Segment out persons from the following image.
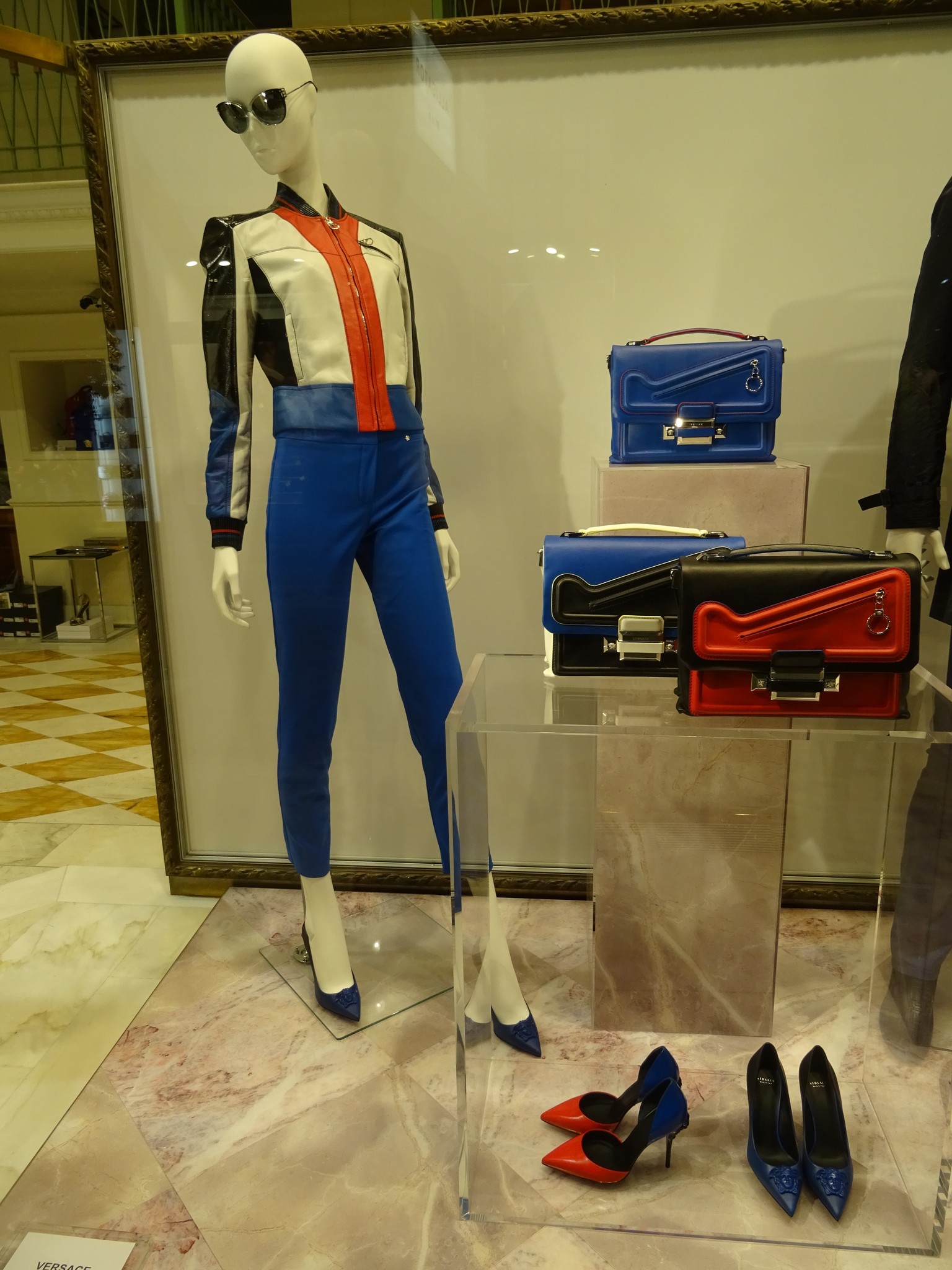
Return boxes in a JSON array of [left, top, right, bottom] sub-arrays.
[[200, 34, 542, 1059], [859, 172, 951, 1053]]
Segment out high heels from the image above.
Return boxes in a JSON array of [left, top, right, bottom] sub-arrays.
[[747, 1043, 799, 1217], [541, 1079, 690, 1186], [799, 1044, 852, 1220], [302, 923, 359, 1022], [541, 1045, 680, 1135], [70, 594, 90, 626], [491, 1001, 542, 1059]]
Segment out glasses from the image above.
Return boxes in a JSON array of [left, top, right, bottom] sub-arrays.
[[218, 81, 318, 134]]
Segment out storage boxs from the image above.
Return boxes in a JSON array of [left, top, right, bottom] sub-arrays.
[[447, 658, 952, 1255], [0, 546, 137, 643]]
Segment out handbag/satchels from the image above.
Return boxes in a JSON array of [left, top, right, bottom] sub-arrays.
[[606, 328, 785, 463], [539, 524, 747, 678], [672, 544, 921, 719]]
